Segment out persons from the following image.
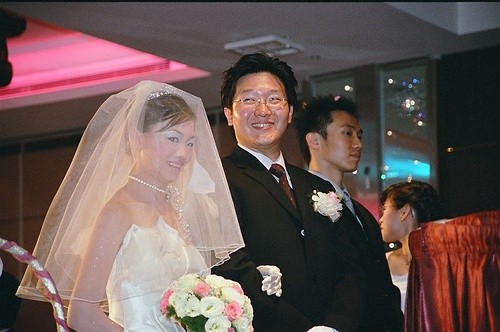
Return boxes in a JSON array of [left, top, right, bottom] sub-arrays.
[[294, 93, 404, 332], [378, 179, 441, 315], [211, 51, 333, 332], [15, 81, 245, 332]]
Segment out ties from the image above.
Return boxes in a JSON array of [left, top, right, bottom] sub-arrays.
[[342, 192, 364, 231], [269, 164, 298, 210]]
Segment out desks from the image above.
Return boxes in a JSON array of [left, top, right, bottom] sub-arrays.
[[405, 210, 500, 332]]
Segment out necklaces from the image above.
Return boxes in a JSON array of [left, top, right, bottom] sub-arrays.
[[127, 176, 167, 193]]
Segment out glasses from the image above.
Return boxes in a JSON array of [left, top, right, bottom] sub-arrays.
[[379, 204, 398, 212], [233, 96, 288, 107]]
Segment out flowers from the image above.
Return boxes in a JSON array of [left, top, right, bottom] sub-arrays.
[[305, 189, 344, 222], [159, 274, 254, 332]]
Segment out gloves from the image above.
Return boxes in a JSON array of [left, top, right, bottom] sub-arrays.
[[256, 265, 283, 298], [307, 325, 338, 332]]
